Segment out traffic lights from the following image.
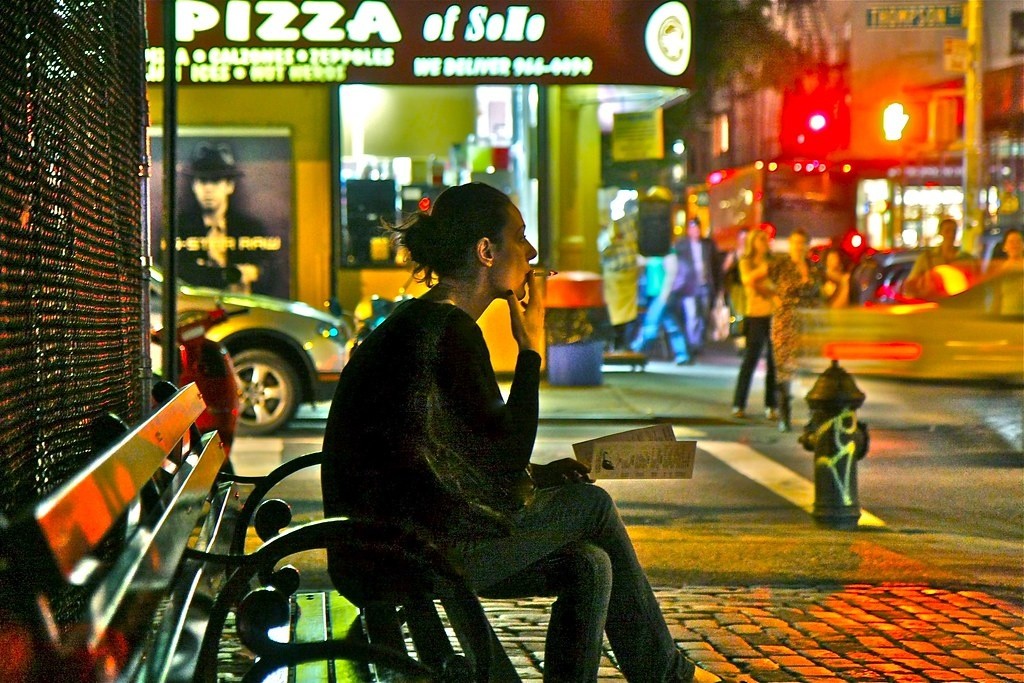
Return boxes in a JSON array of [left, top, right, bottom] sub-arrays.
[[875, 91, 926, 150], [781, 82, 852, 152]]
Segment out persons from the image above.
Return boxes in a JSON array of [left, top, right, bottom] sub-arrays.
[[175, 142, 275, 296], [996, 229, 1024, 316], [321, 183, 723, 683], [748, 229, 845, 431], [731, 228, 777, 419], [823, 249, 860, 309], [601, 198, 748, 365], [902, 219, 965, 296]]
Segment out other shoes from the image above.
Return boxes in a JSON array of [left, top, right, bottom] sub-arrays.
[[765, 409, 778, 420], [733, 407, 744, 417]]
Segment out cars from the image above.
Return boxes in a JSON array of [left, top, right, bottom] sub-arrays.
[[146, 264, 353, 436]]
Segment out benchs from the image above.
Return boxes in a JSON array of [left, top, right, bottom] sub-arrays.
[[12, 382, 493, 683]]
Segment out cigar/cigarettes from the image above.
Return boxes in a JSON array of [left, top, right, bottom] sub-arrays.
[[535, 271, 558, 276]]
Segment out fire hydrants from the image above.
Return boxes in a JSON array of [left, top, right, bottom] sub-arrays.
[[797, 360, 870, 532]]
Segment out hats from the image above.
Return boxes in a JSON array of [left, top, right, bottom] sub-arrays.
[[176, 142, 246, 178]]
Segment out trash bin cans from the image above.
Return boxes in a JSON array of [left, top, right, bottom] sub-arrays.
[[545, 304, 610, 387]]
